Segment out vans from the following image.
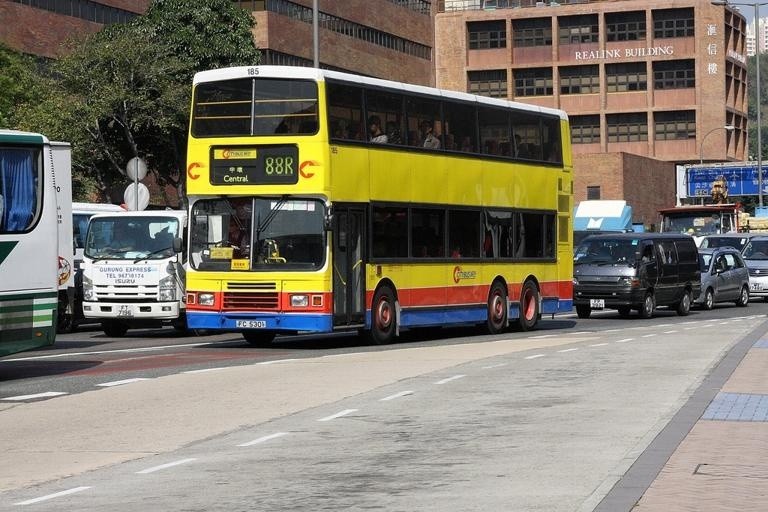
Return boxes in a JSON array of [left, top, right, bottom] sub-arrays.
[[699, 232, 768, 265], [572, 233, 701, 318], [741, 236, 768, 302]]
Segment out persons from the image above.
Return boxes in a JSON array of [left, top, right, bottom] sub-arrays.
[[331, 116, 400, 146], [224, 222, 251, 253], [109, 224, 137, 252], [420, 120, 440, 150]]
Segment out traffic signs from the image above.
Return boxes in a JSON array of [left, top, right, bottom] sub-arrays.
[[742, 167, 768, 195], [688, 168, 741, 197]]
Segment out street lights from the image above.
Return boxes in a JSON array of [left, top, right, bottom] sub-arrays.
[[697, 125, 735, 205], [749, 153, 763, 214], [711, 0, 768, 210]]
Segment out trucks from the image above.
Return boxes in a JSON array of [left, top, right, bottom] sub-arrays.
[[49, 141, 74, 292], [570, 199, 647, 262], [80, 209, 224, 337]]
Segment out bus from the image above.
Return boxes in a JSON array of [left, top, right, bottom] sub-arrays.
[[0, 128, 60, 359], [59, 201, 127, 331], [185, 64, 575, 345]]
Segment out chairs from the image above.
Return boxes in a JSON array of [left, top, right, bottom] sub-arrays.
[[407, 128, 539, 158]]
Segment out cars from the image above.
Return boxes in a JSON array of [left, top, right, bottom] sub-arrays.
[[692, 246, 750, 308]]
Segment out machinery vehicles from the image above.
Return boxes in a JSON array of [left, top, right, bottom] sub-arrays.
[[656, 174, 768, 246]]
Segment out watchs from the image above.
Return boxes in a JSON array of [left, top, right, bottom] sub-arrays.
[[512, 133, 528, 159]]
